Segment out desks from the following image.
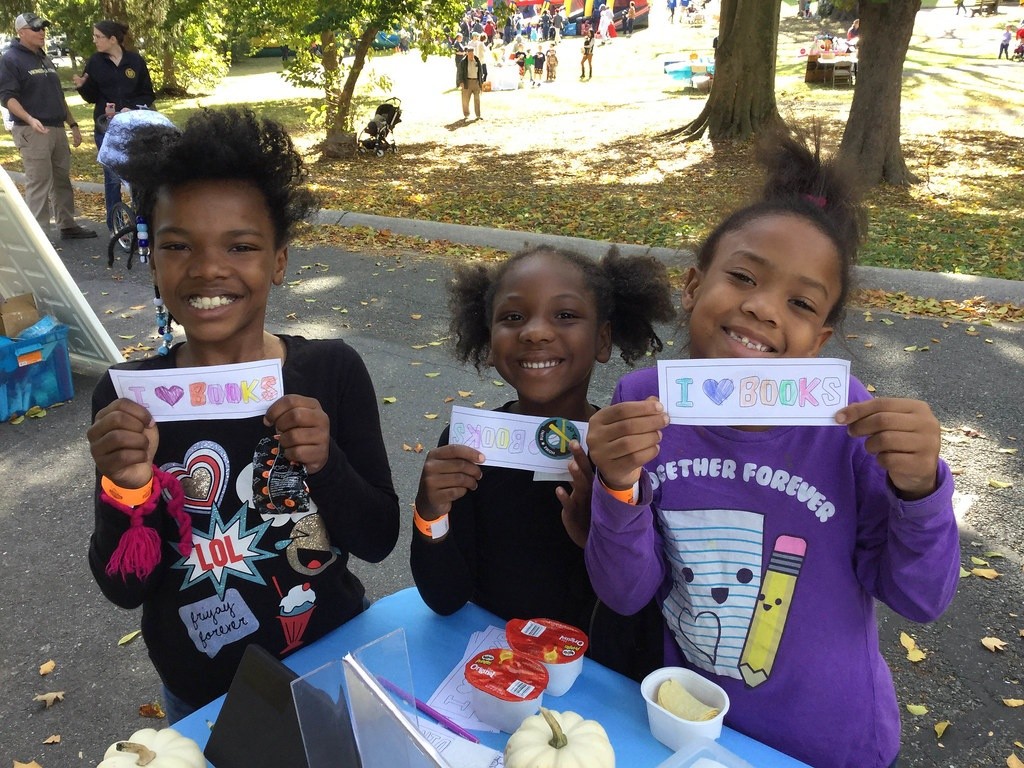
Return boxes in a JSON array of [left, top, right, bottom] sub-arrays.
[[167, 583, 809, 768]]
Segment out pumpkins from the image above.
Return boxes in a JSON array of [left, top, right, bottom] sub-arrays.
[[96, 728, 207, 768], [503, 705, 616, 768]]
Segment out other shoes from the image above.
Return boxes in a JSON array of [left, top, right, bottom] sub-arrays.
[[61, 226, 96, 239]]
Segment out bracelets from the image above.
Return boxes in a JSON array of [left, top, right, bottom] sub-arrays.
[[415, 504, 450, 541], [101, 475, 154, 505], [70, 122, 79, 129], [598, 474, 640, 505]]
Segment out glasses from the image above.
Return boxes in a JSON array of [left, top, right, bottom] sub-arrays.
[[93, 35, 113, 39], [21, 26, 44, 32]]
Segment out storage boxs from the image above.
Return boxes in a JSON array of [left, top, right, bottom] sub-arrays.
[[0, 324, 76, 425], [0, 292, 40, 339]]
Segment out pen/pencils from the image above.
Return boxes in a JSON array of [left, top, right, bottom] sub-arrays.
[[377, 676, 480, 744]]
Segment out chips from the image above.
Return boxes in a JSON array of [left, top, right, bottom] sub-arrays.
[[656, 678, 719, 723]]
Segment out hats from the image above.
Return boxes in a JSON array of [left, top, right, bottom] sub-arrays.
[[15, 13, 50, 32]]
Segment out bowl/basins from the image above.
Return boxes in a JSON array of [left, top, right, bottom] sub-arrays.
[[503, 618, 589, 698], [463, 649, 552, 740], [641, 666, 730, 755]]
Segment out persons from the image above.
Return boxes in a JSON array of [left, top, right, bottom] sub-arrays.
[[805, 1, 811, 17], [584, 122, 962, 768], [73, 21, 157, 239], [847, 19, 859, 79], [410, 245, 678, 682], [88, 108, 402, 725], [454, 2, 636, 120], [0, 13, 98, 241], [667, 0, 700, 24]]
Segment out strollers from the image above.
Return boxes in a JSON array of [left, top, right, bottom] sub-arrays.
[[1011, 39, 1024, 62], [96, 110, 183, 253], [356, 97, 402, 157]]
